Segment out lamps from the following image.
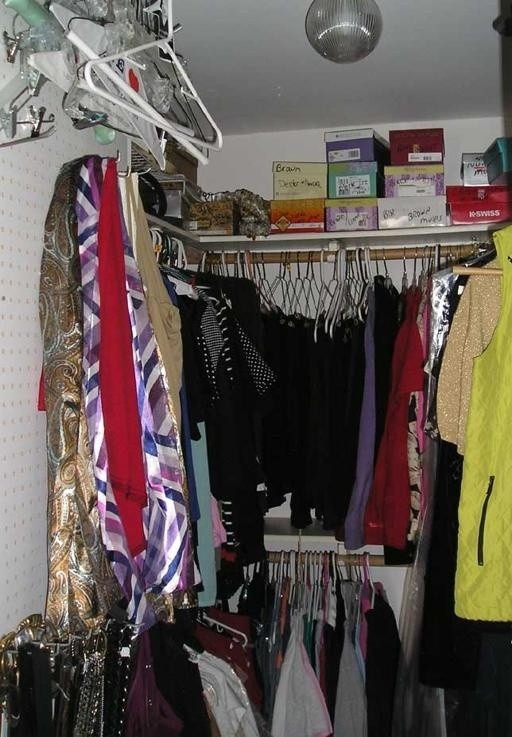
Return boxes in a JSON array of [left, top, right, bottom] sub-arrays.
[[302, 2, 382, 64]]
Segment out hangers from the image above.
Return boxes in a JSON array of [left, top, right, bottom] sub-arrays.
[[260, 541, 373, 665], [1, 591, 249, 675], [17, 0, 222, 164]]
